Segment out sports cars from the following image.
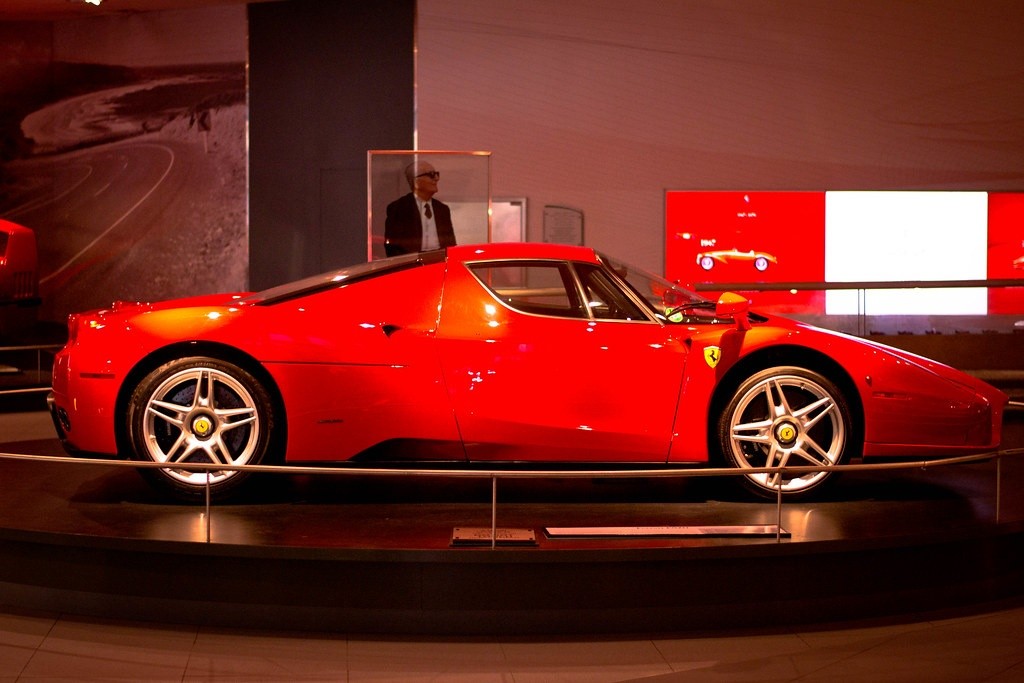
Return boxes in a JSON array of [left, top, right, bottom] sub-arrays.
[[695, 247, 778, 273], [48, 241, 1010, 505]]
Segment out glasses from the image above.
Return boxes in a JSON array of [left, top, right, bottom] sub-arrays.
[[414, 171, 440, 179]]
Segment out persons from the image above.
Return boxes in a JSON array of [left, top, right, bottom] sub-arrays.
[[383, 160, 458, 258]]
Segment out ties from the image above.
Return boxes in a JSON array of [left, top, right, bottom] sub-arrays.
[[423, 203, 432, 219]]
